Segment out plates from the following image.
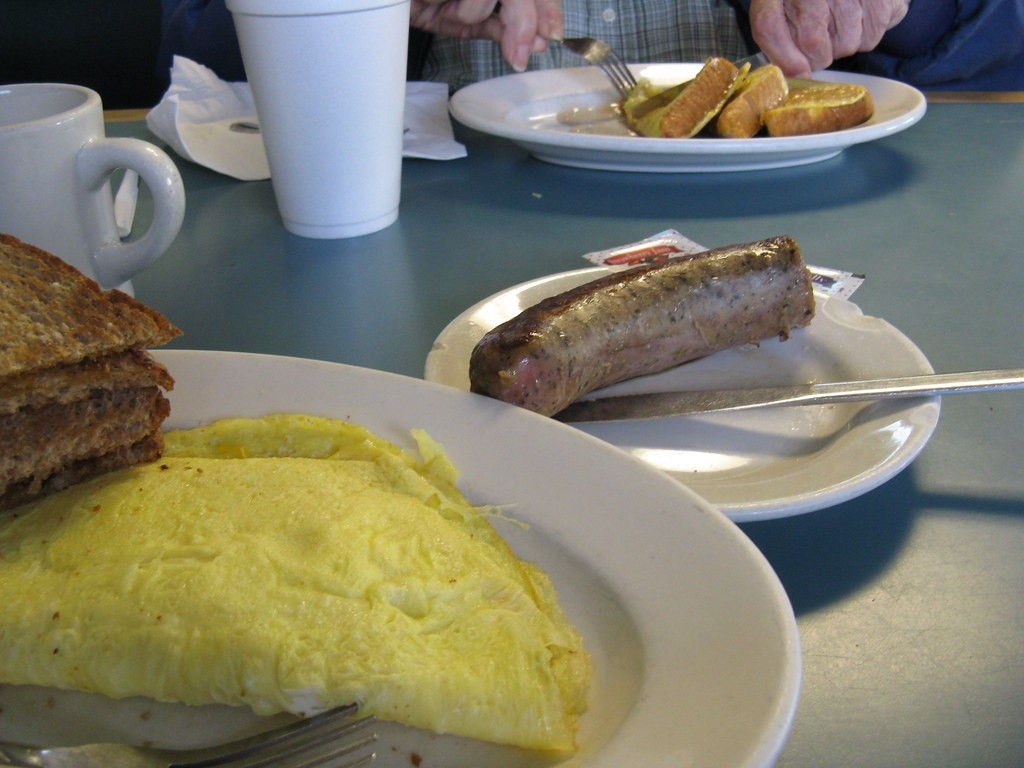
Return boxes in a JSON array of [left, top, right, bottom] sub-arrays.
[[0, 350, 803, 768], [448, 59, 928, 172], [426, 264, 942, 523]]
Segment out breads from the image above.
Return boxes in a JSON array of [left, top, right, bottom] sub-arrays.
[[1, 234, 185, 524]]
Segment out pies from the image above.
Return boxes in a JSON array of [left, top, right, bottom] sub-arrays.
[[1, 413, 593, 753]]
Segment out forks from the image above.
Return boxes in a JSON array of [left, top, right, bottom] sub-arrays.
[[0, 703, 379, 768], [490, 4, 638, 99]]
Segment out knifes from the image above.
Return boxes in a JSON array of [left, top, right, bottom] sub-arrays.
[[551, 365, 1024, 421], [633, 52, 770, 118]]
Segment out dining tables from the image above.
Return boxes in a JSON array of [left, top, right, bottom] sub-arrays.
[[0, 90, 1022, 768]]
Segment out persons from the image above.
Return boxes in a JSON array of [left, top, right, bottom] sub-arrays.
[[139, 0, 1024, 106]]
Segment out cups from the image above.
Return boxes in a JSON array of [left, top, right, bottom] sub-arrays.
[[1, 83, 182, 327], [226, 0, 415, 237]]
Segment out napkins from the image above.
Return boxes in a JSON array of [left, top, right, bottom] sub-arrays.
[[145, 51, 467, 180]]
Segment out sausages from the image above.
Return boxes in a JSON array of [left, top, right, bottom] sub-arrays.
[[467, 233, 816, 418]]
